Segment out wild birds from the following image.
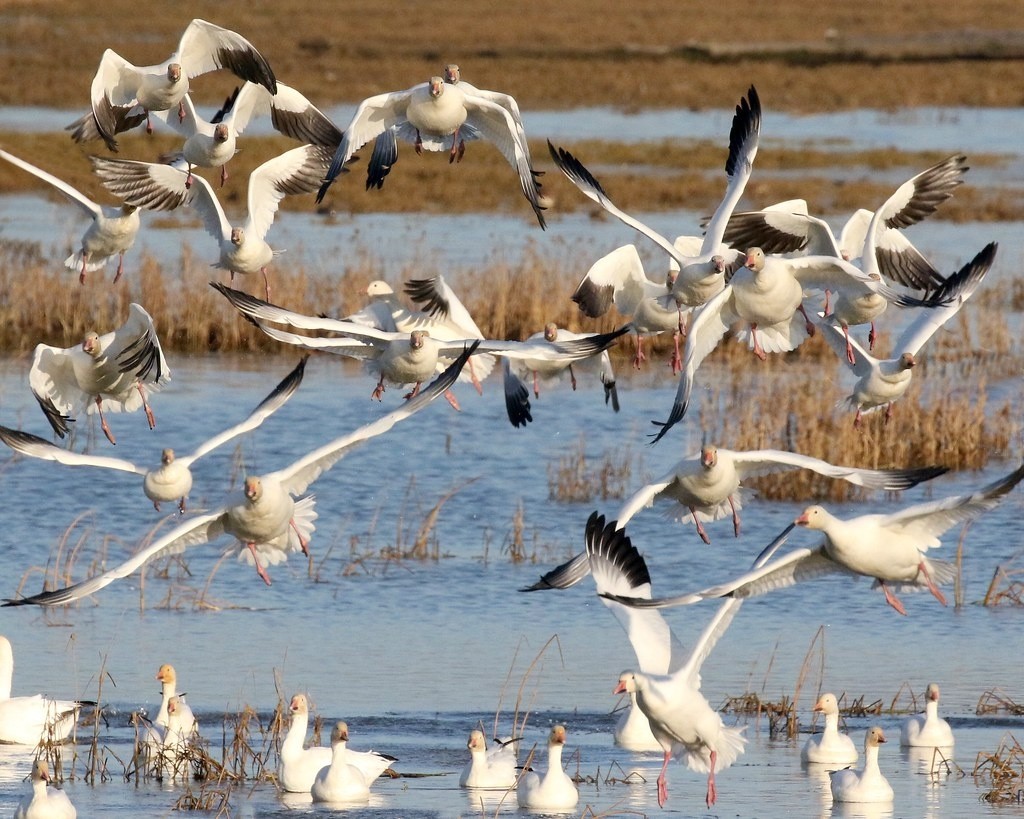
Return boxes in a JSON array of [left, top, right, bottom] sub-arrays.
[[2, 19, 1023, 615], [279, 693, 401, 804], [827, 726, 894, 804], [141, 663, 204, 779], [899, 682, 955, 747], [584, 511, 805, 810], [513, 723, 578, 810], [801, 691, 859, 766], [458, 730, 525, 791], [13, 759, 76, 819], [0, 636, 98, 748]]
